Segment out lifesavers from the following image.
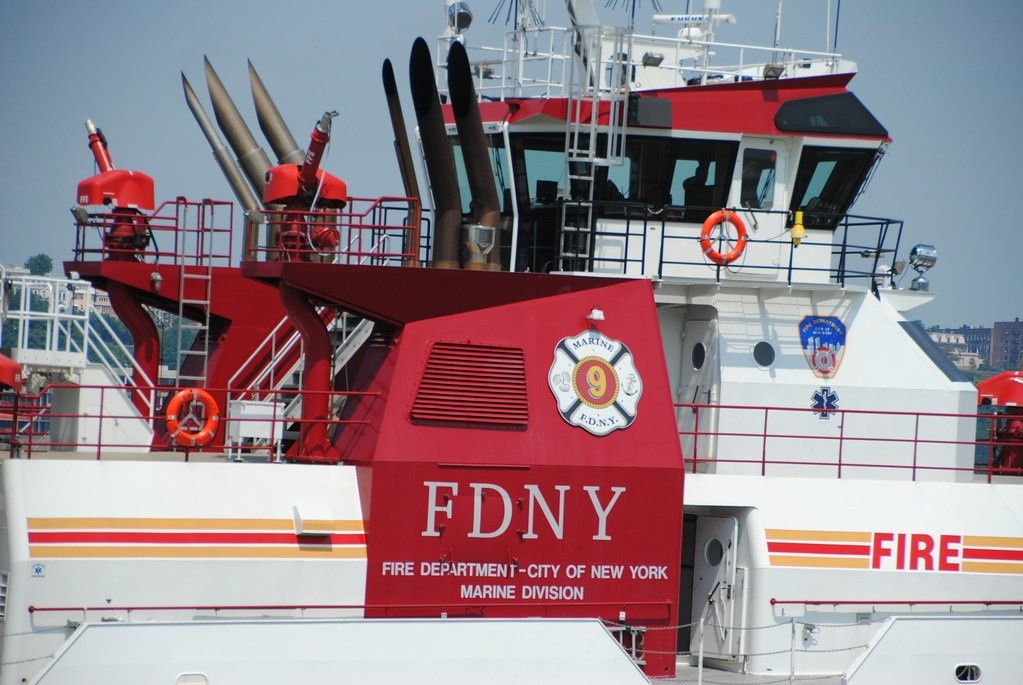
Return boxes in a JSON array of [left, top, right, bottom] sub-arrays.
[[167, 389, 220, 446], [701, 208, 747, 265]]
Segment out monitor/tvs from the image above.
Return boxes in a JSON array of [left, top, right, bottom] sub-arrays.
[[537, 180, 558, 204]]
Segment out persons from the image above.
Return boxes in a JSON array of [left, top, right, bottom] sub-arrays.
[[1000, 407, 1023, 468]]
